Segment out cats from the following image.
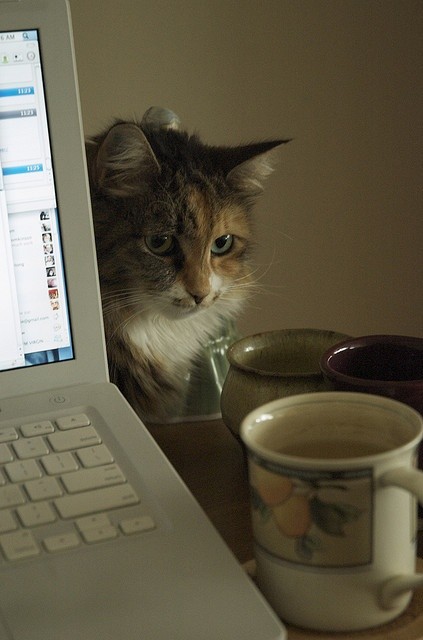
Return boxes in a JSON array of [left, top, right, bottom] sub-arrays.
[[84, 116, 291, 433]]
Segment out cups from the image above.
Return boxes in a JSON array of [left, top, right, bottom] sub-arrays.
[[318, 333, 422, 421], [239, 392, 422, 634], [218, 327, 357, 454]]
